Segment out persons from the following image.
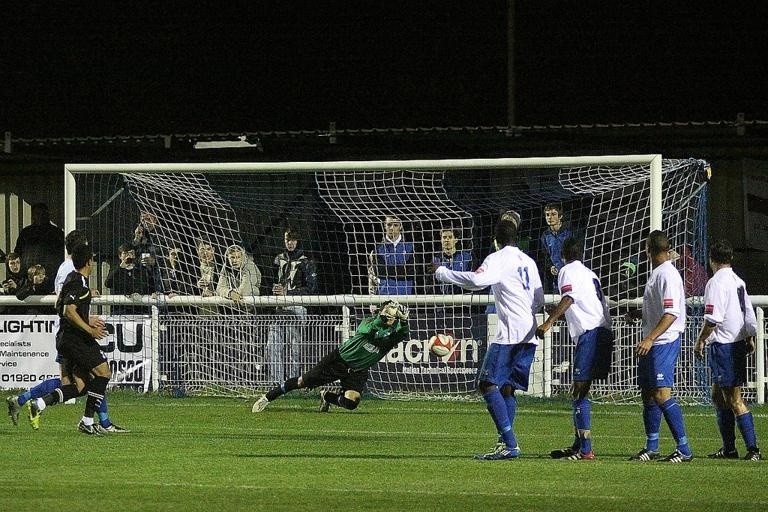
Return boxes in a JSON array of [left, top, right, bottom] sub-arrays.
[[535, 236, 612, 460], [542, 202, 583, 392], [369, 215, 419, 391], [470, 210, 530, 397], [7, 231, 131, 434], [1, 204, 262, 385], [694, 240, 761, 462], [630, 230, 693, 463], [433, 220, 544, 459], [674, 236, 710, 401], [431, 228, 473, 389], [28, 247, 110, 436], [268, 231, 317, 387], [253, 301, 408, 413]]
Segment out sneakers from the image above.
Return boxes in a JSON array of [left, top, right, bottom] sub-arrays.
[[563, 451, 596, 461], [657, 451, 694, 463], [77, 419, 107, 435], [27, 397, 42, 430], [319, 390, 330, 413], [551, 446, 578, 457], [104, 424, 130, 433], [627, 448, 660, 461], [474, 442, 522, 461], [7, 394, 21, 426], [706, 448, 739, 459], [252, 394, 270, 413], [745, 449, 761, 461]]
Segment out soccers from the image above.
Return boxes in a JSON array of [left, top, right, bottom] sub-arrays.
[[428, 334, 451, 357]]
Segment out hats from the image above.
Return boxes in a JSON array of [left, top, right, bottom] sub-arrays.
[[284, 231, 300, 240], [501, 210, 520, 231]]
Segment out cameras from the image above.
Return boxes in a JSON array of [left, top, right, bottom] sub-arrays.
[[3, 280, 10, 287]]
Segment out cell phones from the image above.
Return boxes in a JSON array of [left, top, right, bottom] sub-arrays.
[[125, 258, 138, 264]]
